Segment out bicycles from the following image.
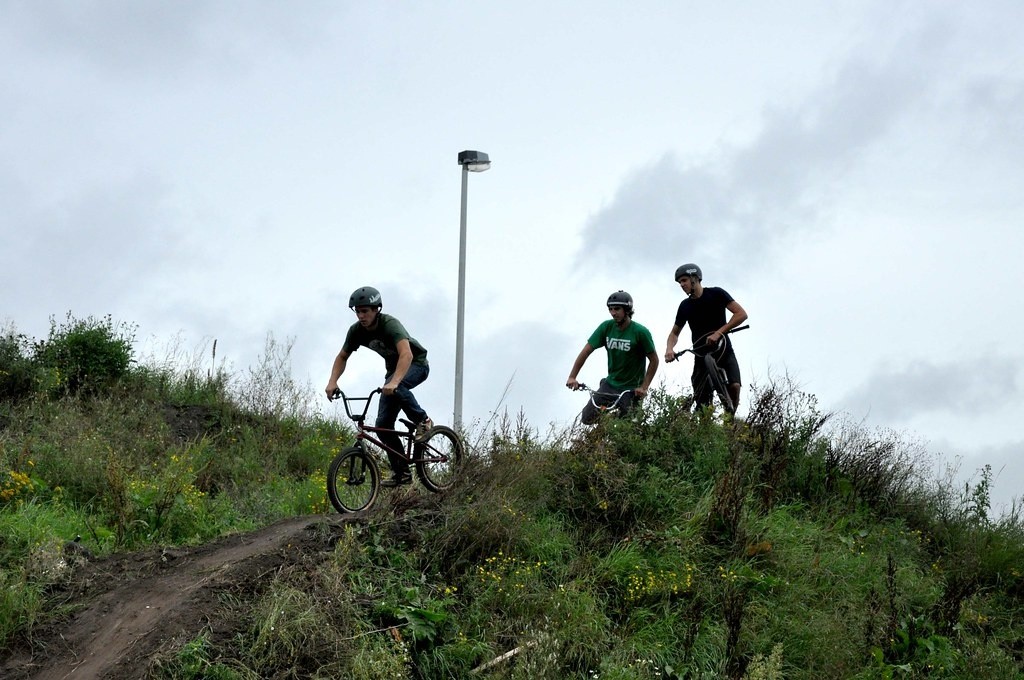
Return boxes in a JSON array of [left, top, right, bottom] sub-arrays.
[[567, 382, 641, 433], [673, 322, 751, 415], [325, 387, 466, 513]]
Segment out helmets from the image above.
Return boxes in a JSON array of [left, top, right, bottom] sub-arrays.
[[607, 291, 634, 308], [674, 264, 702, 282], [349, 286, 382, 312]]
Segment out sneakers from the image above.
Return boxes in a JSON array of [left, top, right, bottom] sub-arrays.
[[413, 417, 434, 444], [380, 469, 414, 488]]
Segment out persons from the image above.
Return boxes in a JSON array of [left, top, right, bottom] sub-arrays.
[[664, 264, 748, 426], [566, 290, 659, 425], [324, 286, 434, 487]]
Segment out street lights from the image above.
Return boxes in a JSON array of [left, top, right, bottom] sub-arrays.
[[450, 150, 493, 444]]
